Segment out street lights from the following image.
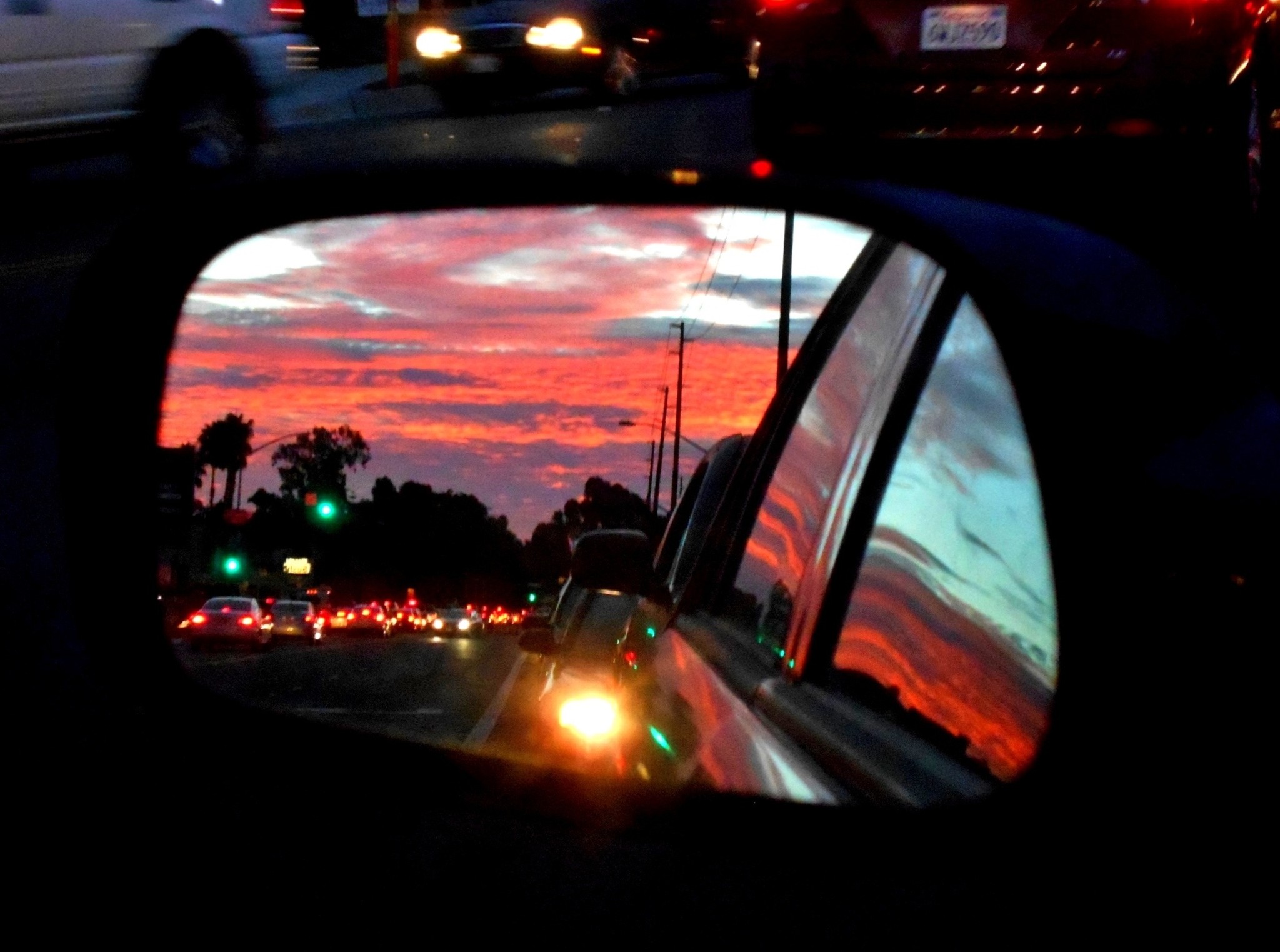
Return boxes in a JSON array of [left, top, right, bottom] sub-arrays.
[[236, 431, 309, 519]]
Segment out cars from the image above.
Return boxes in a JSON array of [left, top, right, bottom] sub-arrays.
[[319, 603, 527, 640], [531, 576, 633, 779], [190, 597, 264, 646], [403, 1, 764, 119], [1, 0, 321, 145], [268, 600, 319, 643]]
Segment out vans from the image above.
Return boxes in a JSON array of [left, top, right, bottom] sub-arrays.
[[568, 526, 653, 595]]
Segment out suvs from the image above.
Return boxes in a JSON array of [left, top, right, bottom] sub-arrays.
[[899, 3, 1278, 203]]
